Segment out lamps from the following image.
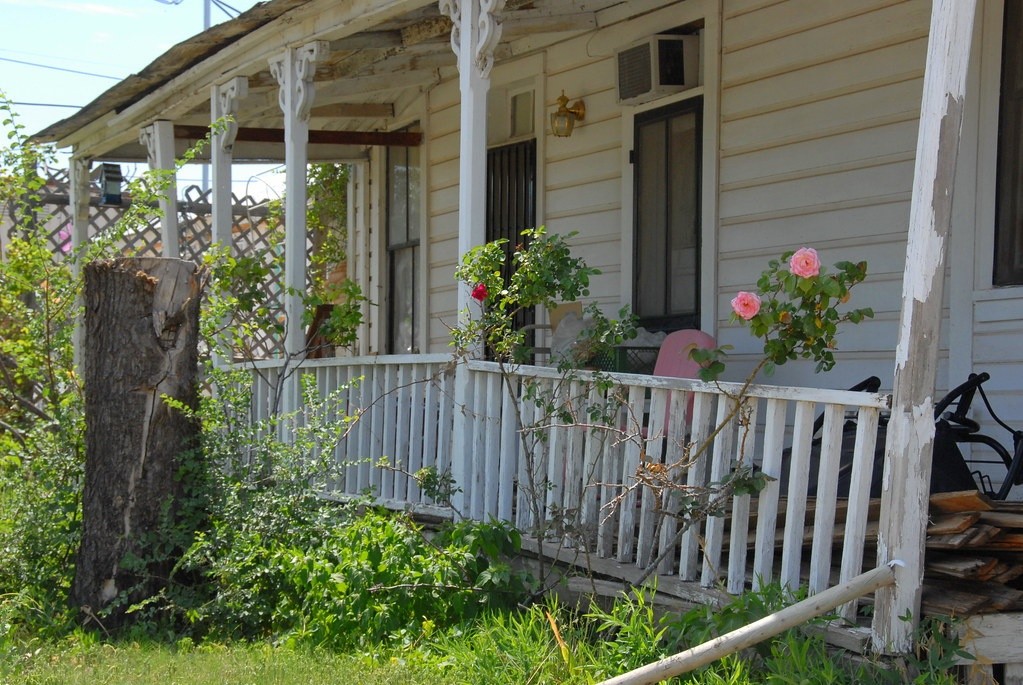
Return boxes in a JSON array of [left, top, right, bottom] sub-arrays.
[[549, 91, 586, 137]]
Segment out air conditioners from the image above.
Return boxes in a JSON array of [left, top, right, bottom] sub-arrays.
[[614, 34, 700, 106]]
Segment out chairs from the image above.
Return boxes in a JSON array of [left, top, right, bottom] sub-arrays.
[[582, 327, 717, 449]]
